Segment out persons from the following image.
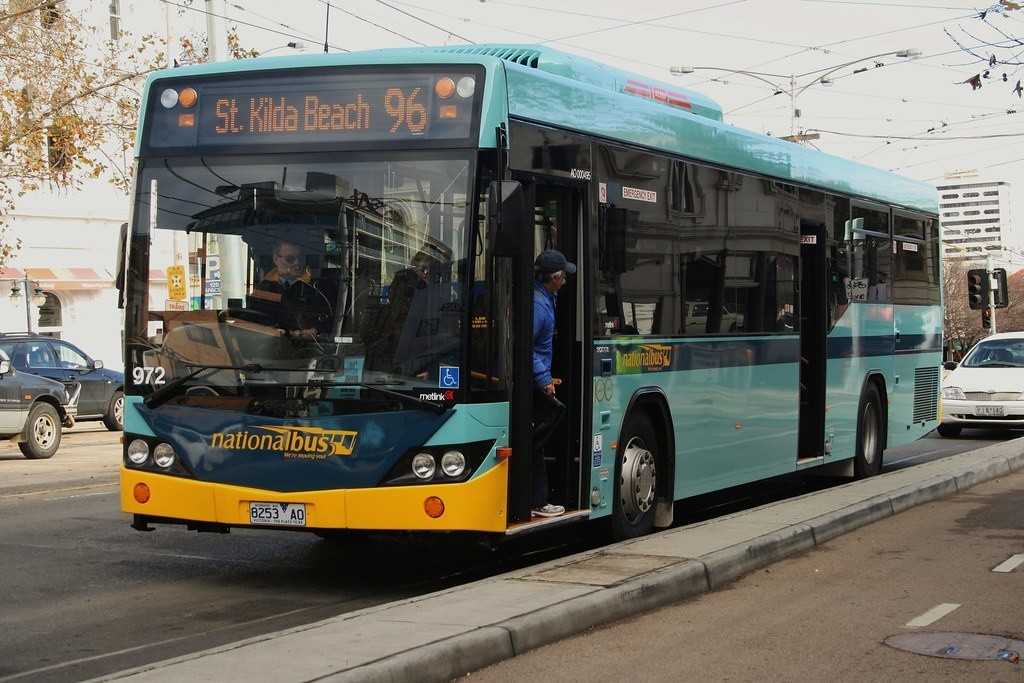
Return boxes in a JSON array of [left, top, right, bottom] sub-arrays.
[[242, 237, 339, 341], [985, 348, 1008, 363], [529, 248, 577, 518]]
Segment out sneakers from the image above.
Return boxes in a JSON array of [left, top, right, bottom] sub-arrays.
[[530, 503, 565, 517]]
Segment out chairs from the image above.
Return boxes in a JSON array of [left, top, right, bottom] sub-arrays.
[[29, 351, 50, 367], [303, 267, 341, 333]]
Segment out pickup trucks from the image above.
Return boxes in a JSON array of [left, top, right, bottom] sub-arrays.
[[0, 347, 76, 460]]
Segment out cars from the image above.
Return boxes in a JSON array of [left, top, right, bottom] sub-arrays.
[[0, 330, 124, 430], [935, 330, 1024, 436]]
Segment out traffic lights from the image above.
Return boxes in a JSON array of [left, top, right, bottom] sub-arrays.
[[982, 308, 990, 327], [966, 269, 990, 310]]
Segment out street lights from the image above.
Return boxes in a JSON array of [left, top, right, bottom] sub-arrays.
[[669, 44, 924, 144], [8, 269, 48, 334]]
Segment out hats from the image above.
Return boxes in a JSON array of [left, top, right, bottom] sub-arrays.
[[534, 250, 576, 275]]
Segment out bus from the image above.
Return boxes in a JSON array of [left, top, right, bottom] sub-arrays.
[[115, 45, 945, 569]]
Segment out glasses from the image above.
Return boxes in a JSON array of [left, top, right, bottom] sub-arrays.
[[277, 255, 307, 264]]
